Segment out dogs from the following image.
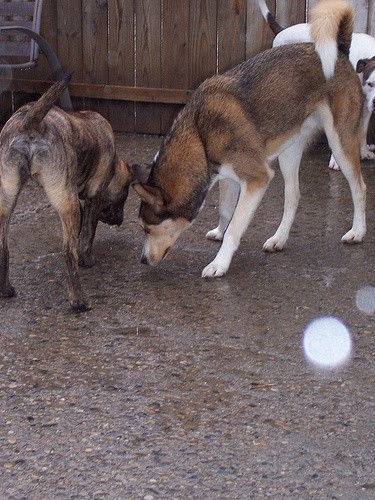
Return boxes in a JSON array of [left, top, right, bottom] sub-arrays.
[[259, 0, 375, 171], [0, 73, 136, 314], [129, 0, 368, 278]]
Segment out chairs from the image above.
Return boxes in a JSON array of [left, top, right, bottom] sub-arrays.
[[0, 0, 74, 113]]
